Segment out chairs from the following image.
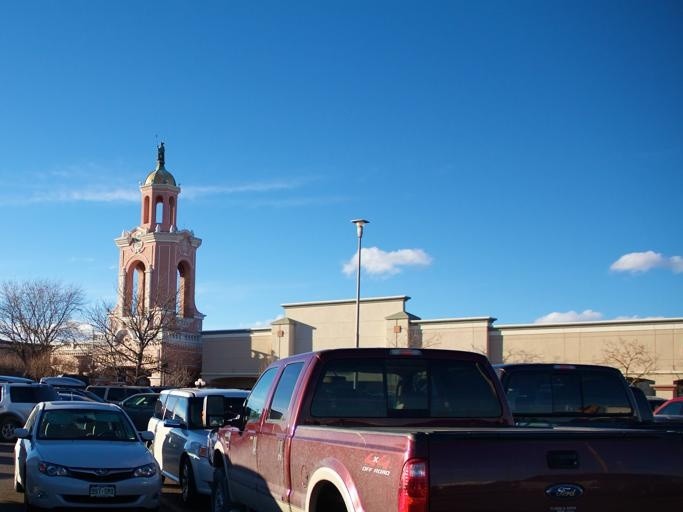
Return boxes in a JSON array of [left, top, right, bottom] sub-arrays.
[[43, 414, 80, 438]]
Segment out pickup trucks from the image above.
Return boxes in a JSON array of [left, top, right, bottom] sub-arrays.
[[208, 346, 681, 512]]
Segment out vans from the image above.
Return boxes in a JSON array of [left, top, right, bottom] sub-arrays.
[[146, 379, 253, 508]]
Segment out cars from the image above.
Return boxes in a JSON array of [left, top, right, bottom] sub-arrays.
[[490, 362, 683, 431], [13, 400, 164, 512], [1, 373, 161, 441]]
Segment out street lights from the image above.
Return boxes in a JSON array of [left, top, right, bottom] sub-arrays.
[[350, 217, 371, 349]]
